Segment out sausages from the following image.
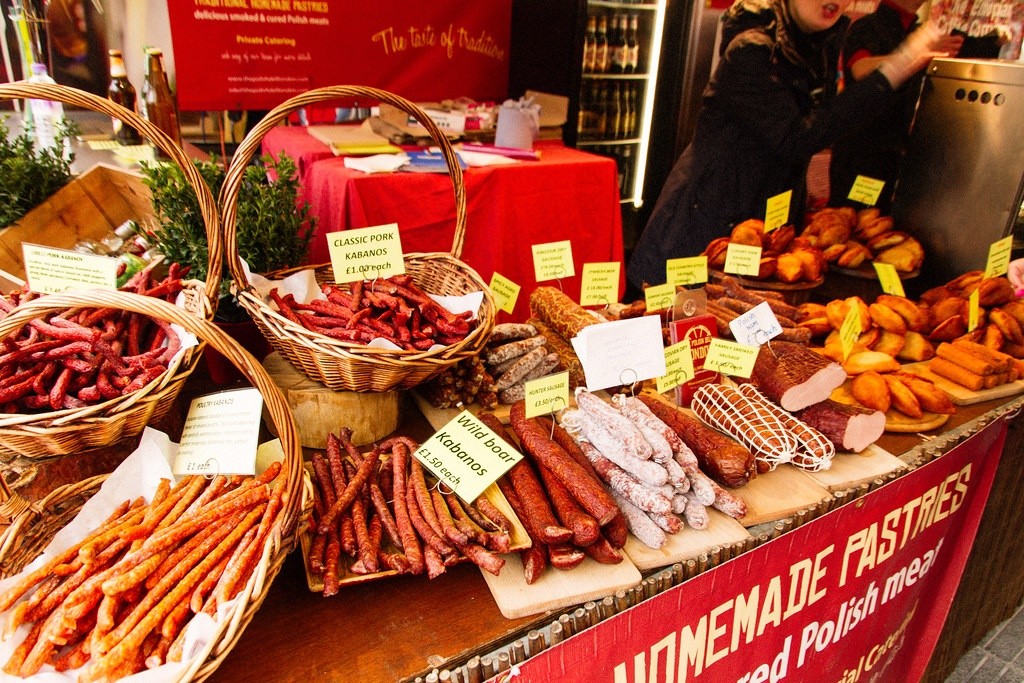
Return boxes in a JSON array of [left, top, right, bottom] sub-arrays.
[[2, 252, 886, 681]]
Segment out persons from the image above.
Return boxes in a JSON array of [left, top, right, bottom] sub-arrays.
[[644, 1, 948, 297]]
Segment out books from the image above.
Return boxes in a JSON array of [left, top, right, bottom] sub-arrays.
[[398, 152, 469, 173], [305, 124, 403, 156]]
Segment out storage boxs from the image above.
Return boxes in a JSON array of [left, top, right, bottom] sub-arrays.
[[0, 162, 196, 298]]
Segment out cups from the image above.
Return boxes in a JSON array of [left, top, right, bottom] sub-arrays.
[[496, 105, 534, 152]]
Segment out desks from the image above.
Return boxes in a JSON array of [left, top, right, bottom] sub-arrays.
[[0, 307, 1023, 683], [261, 124, 469, 218], [290, 142, 626, 323]]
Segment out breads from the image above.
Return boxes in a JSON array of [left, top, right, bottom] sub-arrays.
[[697, 206, 1023, 418]]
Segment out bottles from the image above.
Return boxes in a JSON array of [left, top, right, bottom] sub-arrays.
[[72, 216, 160, 285], [139, 43, 185, 162], [26, 62, 74, 155], [577, 78, 640, 140], [107, 49, 141, 146], [577, 5, 641, 73], [591, 149, 632, 200]]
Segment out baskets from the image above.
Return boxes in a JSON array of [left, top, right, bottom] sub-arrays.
[[218, 83, 499, 395], [0, 81, 224, 462], [0, 289, 315, 683]]
